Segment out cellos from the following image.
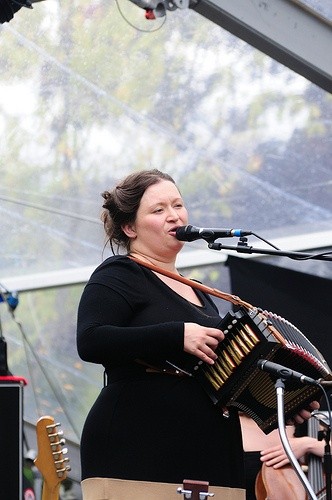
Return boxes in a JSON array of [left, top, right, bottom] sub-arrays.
[[253, 406, 332, 500]]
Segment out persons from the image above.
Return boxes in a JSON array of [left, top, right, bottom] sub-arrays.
[[77, 168, 320, 500], [258, 434, 332, 470]]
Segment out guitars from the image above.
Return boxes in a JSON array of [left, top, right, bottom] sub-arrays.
[[32, 416, 71, 499]]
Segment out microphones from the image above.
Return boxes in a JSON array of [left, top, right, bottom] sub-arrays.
[[175, 225, 253, 241], [256, 358, 316, 385]]
